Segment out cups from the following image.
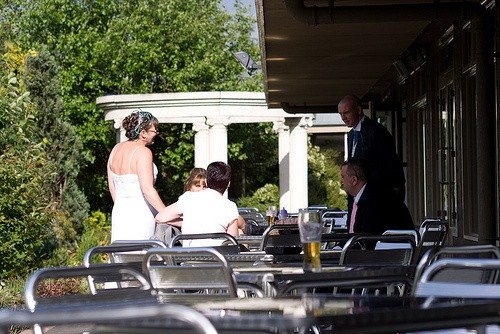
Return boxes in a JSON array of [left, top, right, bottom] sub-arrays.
[[266, 210, 276, 226], [298, 208, 323, 273]]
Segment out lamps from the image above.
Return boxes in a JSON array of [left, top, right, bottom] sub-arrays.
[[232, 52, 263, 75], [393, 60, 416, 83]]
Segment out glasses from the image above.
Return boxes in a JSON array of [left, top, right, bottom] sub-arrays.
[[146, 129, 159, 135]]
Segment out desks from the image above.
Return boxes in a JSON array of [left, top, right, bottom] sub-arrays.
[[165, 294, 414, 316], [235, 232, 363, 250], [237, 267, 410, 286]]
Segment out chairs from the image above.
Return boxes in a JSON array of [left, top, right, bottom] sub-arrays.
[[0, 205, 500, 334]]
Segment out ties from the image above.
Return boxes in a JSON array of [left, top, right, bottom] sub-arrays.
[[348, 201, 359, 233], [352, 130, 359, 158]]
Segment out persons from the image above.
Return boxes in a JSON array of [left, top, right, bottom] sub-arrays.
[[339, 95, 395, 195], [327, 159, 416, 248], [107, 111, 166, 254], [154, 162, 246, 248]]
